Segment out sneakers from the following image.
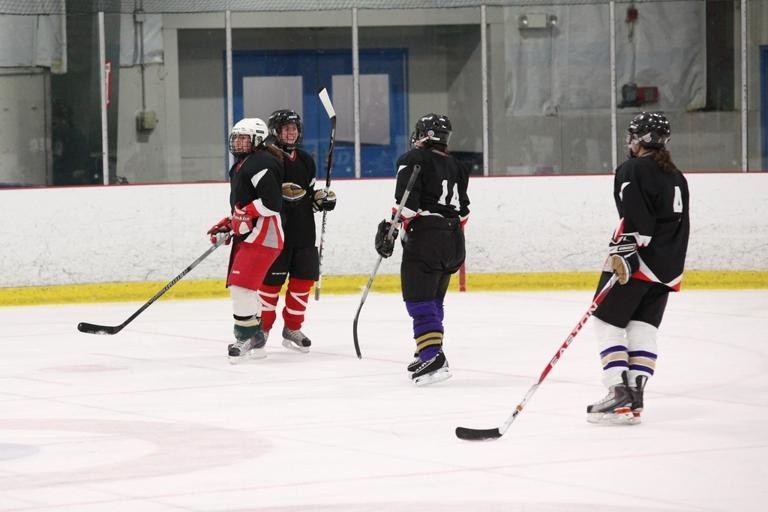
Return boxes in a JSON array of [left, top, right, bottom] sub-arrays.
[[630, 387, 644, 413], [252, 330, 269, 347], [407, 354, 424, 372], [588, 384, 631, 412], [412, 349, 449, 379], [282, 326, 311, 347], [228, 337, 255, 356]]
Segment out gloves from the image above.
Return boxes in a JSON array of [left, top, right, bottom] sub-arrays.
[[207, 217, 233, 246], [610, 234, 640, 273], [282, 183, 307, 202], [231, 202, 253, 236], [311, 189, 336, 213], [375, 219, 398, 258]]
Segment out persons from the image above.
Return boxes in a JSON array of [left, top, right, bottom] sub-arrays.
[[206, 117, 286, 364], [586, 112, 690, 425], [375, 111, 471, 385], [254, 109, 336, 352]]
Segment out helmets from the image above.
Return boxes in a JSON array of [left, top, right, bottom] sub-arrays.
[[624, 112, 671, 158], [409, 114, 453, 149], [228, 117, 269, 158], [267, 110, 303, 151]]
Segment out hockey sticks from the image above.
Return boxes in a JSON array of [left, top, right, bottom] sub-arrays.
[[354, 165, 420, 358], [314, 88, 336, 300], [455, 273, 619, 441], [77, 232, 230, 334]]
